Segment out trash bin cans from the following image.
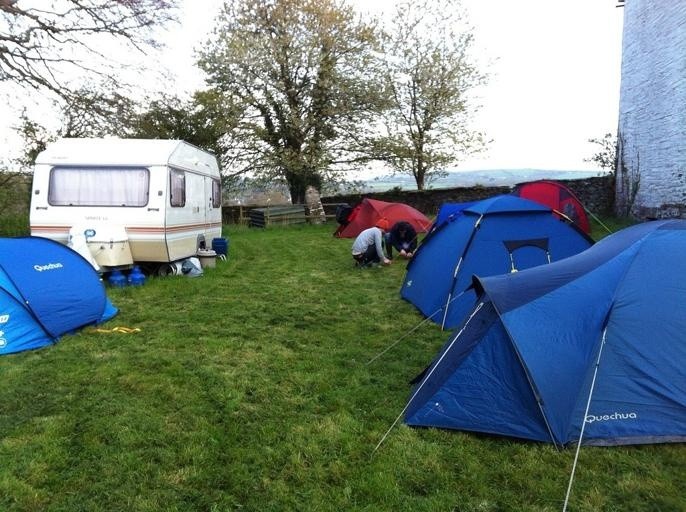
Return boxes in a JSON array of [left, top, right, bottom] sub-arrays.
[[196, 237, 228, 269]]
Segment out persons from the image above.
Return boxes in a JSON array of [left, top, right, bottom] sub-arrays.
[[384, 221, 418, 258], [352, 225, 391, 267]]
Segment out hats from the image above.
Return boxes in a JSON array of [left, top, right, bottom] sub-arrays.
[[376, 218, 389, 230]]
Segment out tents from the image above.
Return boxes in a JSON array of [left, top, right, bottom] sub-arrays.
[[332, 198, 433, 238], [398, 194, 594, 331], [436, 199, 479, 227], [405, 216, 686, 452], [519, 179, 591, 234], [1, 235, 120, 357]]
